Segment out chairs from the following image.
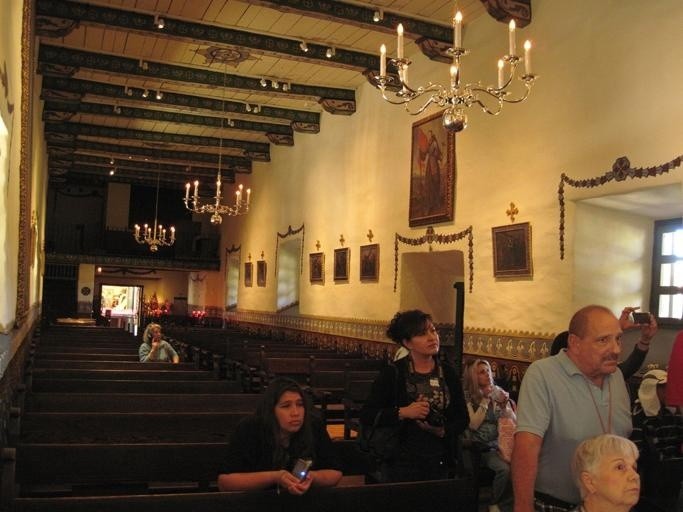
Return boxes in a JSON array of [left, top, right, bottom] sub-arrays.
[[627, 412, 683, 512]]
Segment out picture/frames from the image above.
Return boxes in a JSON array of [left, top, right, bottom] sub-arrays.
[[408, 106, 456, 226], [492, 222, 533, 278], [309, 252, 325, 281], [245, 261, 254, 287], [256, 260, 267, 287], [334, 247, 350, 280], [360, 244, 380, 280]]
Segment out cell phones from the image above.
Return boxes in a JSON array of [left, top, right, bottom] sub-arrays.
[[632, 309, 652, 328]]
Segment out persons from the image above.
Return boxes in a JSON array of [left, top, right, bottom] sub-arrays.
[[457, 359, 517, 512], [572, 434, 642, 512], [216, 377, 342, 498], [665, 330, 682, 417], [418, 129, 443, 216], [510, 303, 634, 511], [139, 322, 180, 363], [356, 309, 470, 485], [549, 305, 659, 382]]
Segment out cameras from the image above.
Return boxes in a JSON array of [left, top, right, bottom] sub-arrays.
[[288, 458, 314, 478]]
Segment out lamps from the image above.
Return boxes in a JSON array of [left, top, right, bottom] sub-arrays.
[[109, 155, 118, 177], [325, 46, 335, 58], [374, 1, 541, 134], [300, 41, 308, 53], [132, 158, 177, 253], [182, 54, 252, 225], [373, 10, 382, 22], [228, 78, 290, 127], [112, 15, 168, 115]]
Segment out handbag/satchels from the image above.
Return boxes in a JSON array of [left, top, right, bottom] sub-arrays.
[[496, 419, 517, 462]]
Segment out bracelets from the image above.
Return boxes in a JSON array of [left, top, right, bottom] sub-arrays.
[[479, 403, 488, 411], [639, 339, 650, 346]]
